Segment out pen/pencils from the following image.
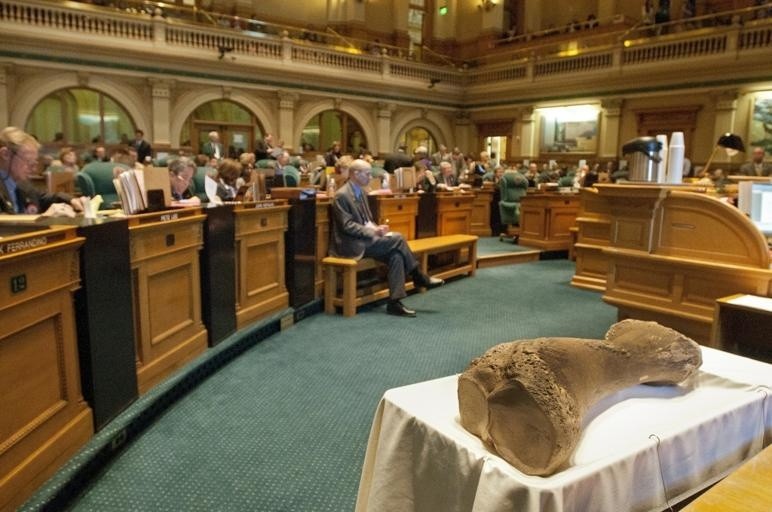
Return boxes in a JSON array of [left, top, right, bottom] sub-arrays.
[[384, 219, 390, 225]]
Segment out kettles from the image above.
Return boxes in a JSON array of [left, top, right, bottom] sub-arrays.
[[620, 136, 663, 184]]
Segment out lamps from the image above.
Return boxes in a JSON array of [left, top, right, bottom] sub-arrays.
[[697, 133, 745, 177]]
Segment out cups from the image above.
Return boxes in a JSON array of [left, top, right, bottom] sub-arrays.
[[82, 200, 100, 217], [654, 130, 685, 184]]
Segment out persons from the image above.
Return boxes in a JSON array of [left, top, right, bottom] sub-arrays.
[[355, 144, 601, 188], [331, 159, 446, 318], [640, 0, 772, 43], [500, 14, 597, 44], [247, 14, 263, 52], [0, 126, 355, 219], [301, 23, 319, 43], [741, 146, 772, 176], [366, 38, 382, 54]]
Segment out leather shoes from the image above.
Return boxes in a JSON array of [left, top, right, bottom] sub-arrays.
[[414, 271, 445, 288], [386, 300, 417, 316]]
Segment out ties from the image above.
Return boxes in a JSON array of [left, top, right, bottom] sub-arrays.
[[359, 194, 370, 222]]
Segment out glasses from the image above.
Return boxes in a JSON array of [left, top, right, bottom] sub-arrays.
[[13, 151, 39, 166]]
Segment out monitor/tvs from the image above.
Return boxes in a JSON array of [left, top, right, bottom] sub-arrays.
[[752, 184, 772, 249]]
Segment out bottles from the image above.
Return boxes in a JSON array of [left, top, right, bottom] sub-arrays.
[[328, 178, 336, 194]]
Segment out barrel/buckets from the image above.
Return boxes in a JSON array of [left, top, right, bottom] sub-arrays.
[[627, 138, 663, 181]]
